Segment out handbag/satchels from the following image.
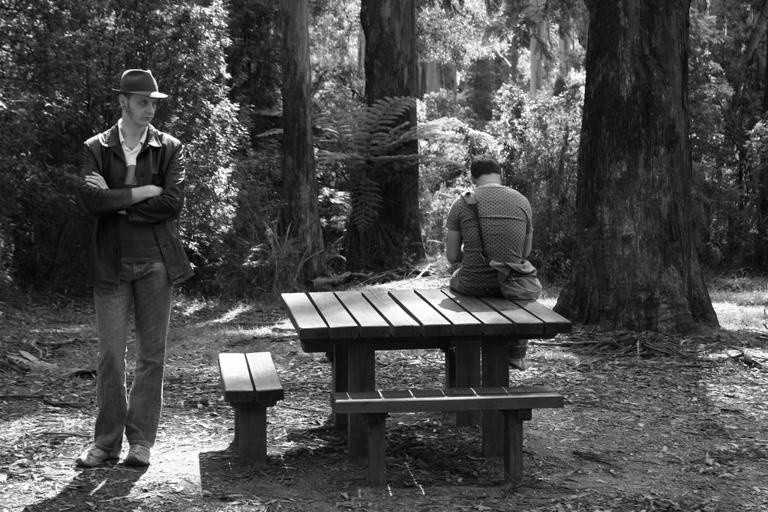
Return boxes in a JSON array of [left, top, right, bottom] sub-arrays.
[[489, 259, 542, 300]]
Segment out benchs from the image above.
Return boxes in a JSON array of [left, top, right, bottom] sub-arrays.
[[218, 351, 284, 467], [331, 386, 564, 487]]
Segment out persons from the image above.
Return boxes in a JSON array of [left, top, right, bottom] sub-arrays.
[[445, 148, 542, 369], [69, 69, 191, 471]]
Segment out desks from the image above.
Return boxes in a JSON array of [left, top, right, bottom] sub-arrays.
[[281, 288, 571, 466]]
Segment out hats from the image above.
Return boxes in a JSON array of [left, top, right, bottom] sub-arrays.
[[112, 69, 169, 99]]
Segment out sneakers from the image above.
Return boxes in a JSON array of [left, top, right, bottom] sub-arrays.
[[76, 446, 119, 466], [509, 357, 527, 371], [127, 445, 151, 465]]
